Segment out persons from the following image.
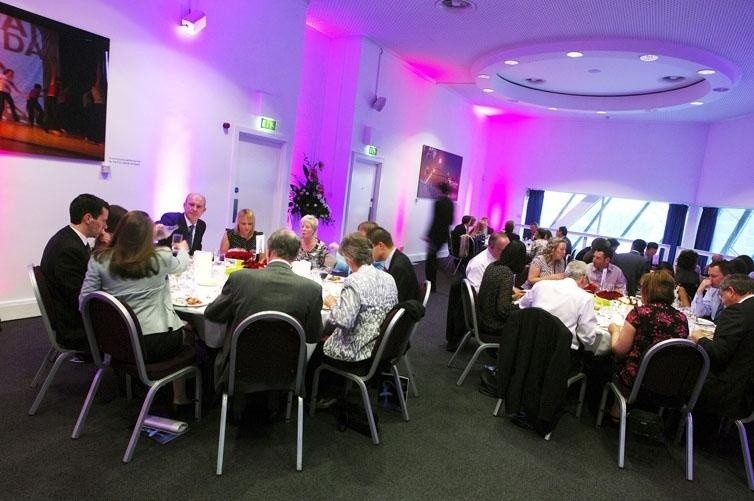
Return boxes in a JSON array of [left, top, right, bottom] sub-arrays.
[[202, 229, 325, 427], [306, 230, 401, 409], [154, 191, 208, 258], [218, 207, 264, 259], [419, 179, 458, 297], [447, 214, 754, 431], [368, 226, 421, 307], [1, 59, 105, 141], [291, 213, 328, 269], [76, 210, 198, 410], [36, 191, 112, 354], [102, 203, 168, 239], [324, 221, 387, 276]]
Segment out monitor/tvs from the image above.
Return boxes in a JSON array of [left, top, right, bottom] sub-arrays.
[[0, 2, 110, 161]]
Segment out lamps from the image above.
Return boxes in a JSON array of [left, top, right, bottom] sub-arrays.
[[178, 0, 206, 36]]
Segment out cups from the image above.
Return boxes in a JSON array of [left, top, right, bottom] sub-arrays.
[[214, 254, 225, 268], [171, 234, 181, 253], [331, 264, 342, 282]]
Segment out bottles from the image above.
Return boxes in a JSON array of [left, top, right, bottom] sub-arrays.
[[154, 225, 177, 241]]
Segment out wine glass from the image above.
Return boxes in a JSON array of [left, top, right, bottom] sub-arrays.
[[318, 267, 329, 285]]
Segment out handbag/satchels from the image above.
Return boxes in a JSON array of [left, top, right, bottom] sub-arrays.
[[480, 367, 499, 398], [626, 410, 665, 439], [439, 258, 455, 273], [335, 401, 378, 437]]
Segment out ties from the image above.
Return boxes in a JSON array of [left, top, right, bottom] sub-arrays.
[[188, 225, 194, 247]]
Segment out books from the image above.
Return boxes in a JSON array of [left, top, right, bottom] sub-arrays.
[[141, 413, 190, 446]]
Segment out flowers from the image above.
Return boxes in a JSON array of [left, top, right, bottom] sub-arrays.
[[287, 154, 337, 224]]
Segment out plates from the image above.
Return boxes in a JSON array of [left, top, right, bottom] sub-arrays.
[[696, 318, 714, 327], [173, 299, 206, 308]]
